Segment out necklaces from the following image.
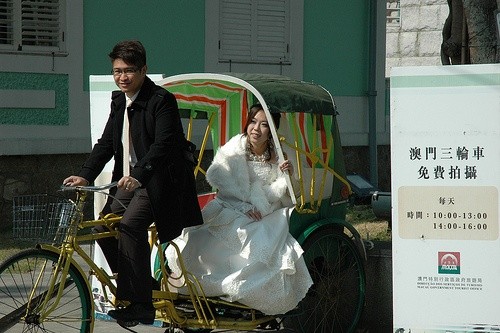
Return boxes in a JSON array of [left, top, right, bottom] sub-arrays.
[[246, 138, 271, 162]]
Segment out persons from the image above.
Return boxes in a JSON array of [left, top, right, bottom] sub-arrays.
[[63, 41, 203, 325], [200, 103, 294, 298]]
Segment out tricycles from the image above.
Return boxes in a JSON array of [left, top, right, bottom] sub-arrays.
[[0, 73, 368, 333]]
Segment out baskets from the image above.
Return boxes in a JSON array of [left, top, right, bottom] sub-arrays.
[[12, 193, 81, 244]]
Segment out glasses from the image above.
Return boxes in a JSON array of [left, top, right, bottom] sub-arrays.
[[111, 67, 142, 75]]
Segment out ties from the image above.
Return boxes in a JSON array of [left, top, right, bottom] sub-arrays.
[[122, 98, 132, 177]]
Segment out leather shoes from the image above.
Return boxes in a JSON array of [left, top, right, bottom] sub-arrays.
[[108, 305, 155, 325]]
